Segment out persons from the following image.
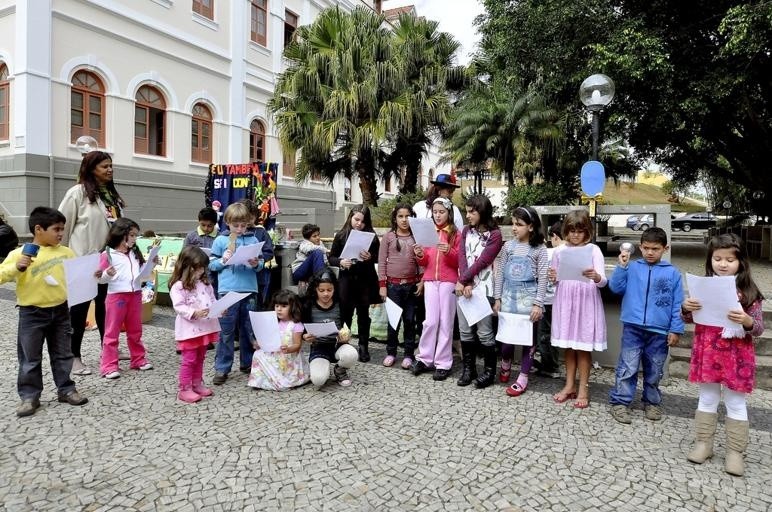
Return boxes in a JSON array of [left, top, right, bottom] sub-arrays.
[[529, 220, 566, 378], [679, 233, 766, 477], [1, 207, 102, 417], [292, 224, 333, 285], [57, 151, 130, 376], [327, 205, 381, 363], [607, 227, 685, 425], [451, 192, 503, 389], [169, 244, 230, 403], [246, 289, 313, 389], [237, 199, 276, 312], [175, 207, 217, 355], [410, 174, 465, 237], [491, 204, 548, 398], [547, 208, 608, 408], [299, 268, 359, 388], [405, 194, 462, 381], [93, 217, 159, 378], [208, 201, 267, 386], [377, 203, 423, 370]]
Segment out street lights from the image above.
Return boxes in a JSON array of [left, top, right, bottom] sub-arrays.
[[706, 207, 712, 222], [752, 191, 765, 222], [722, 200, 732, 231], [578, 75, 616, 243]]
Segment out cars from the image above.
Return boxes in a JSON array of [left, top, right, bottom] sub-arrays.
[[637, 212, 681, 231], [627, 217, 639, 231], [671, 212, 718, 232]]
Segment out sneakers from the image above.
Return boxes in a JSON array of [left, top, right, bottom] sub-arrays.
[[239, 366, 251, 373], [176, 382, 203, 404], [333, 364, 351, 387], [610, 403, 631, 424], [432, 369, 452, 381], [139, 362, 154, 371], [412, 361, 430, 377], [193, 377, 212, 397], [105, 371, 121, 379], [401, 357, 413, 370], [212, 371, 228, 384], [382, 355, 396, 367], [645, 402, 662, 420]]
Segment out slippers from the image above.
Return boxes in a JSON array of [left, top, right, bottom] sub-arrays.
[[553, 389, 577, 403], [574, 394, 589, 408]]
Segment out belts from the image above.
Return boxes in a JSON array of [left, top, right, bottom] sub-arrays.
[[386, 273, 425, 286]]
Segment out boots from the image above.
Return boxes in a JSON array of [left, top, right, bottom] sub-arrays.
[[470, 343, 496, 389], [357, 317, 371, 362], [724, 414, 749, 476], [456, 340, 478, 386], [687, 408, 719, 464]]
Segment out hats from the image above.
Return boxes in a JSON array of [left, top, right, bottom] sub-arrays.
[[429, 167, 461, 188]]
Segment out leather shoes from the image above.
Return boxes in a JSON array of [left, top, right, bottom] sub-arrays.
[[16, 397, 40, 416], [99, 349, 132, 360], [71, 364, 92, 375], [57, 390, 89, 406], [499, 364, 511, 383], [506, 381, 528, 396]]
